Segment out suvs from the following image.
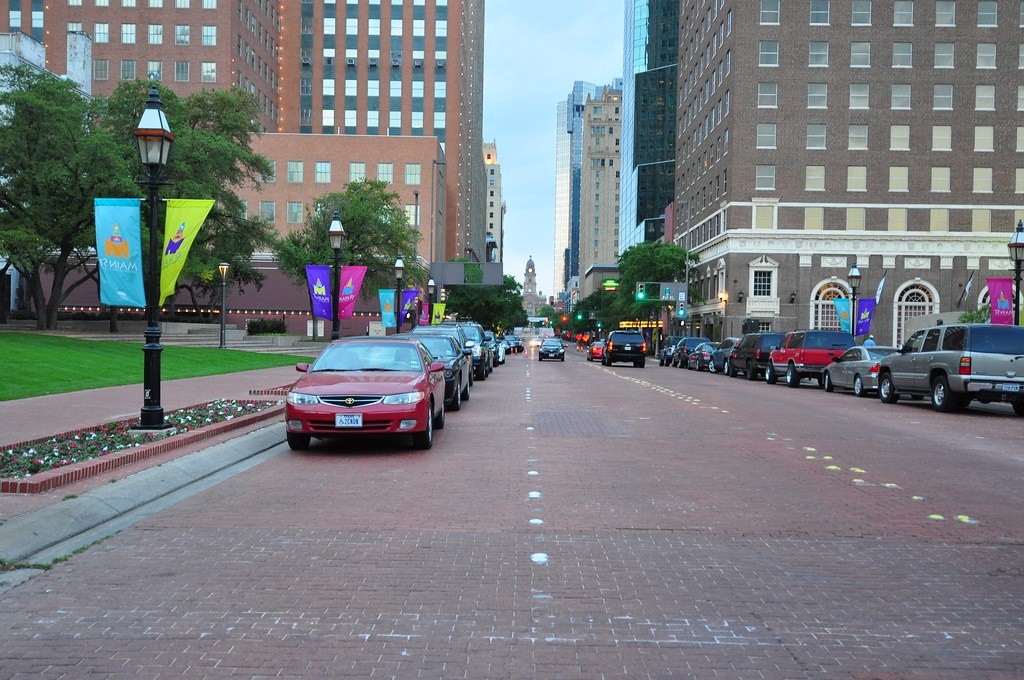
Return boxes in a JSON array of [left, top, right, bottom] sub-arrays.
[[660, 336, 713, 368], [765, 328, 857, 387], [877, 323, 1024, 416], [602, 330, 647, 368], [729, 332, 786, 380]]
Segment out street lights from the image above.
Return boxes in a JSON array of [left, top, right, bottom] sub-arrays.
[[1008, 219, 1024, 325], [132, 84, 177, 429], [427, 276, 435, 324], [847, 262, 862, 338], [219, 262, 230, 348], [394, 254, 406, 334], [328, 207, 346, 341]]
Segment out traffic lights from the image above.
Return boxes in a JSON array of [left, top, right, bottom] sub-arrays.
[[638, 283, 644, 299], [679, 303, 684, 315]]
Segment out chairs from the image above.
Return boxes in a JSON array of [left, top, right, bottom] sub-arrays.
[[431, 340, 449, 356], [333, 350, 359, 370], [394, 348, 413, 366]]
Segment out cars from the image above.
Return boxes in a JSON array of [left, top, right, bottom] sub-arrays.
[[687, 342, 722, 371], [285, 335, 446, 451], [392, 333, 472, 410], [823, 346, 925, 399], [411, 322, 524, 385], [708, 337, 747, 375], [586, 341, 605, 361], [537, 339, 566, 361], [530, 337, 568, 347]]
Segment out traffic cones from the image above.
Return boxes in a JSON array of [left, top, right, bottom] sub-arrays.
[[511, 346, 528, 358], [576, 346, 583, 352]]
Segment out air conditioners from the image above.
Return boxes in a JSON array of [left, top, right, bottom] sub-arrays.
[[392, 59, 399, 66], [414, 60, 422, 66], [369, 57, 377, 66], [302, 57, 310, 64], [436, 61, 444, 67], [347, 59, 355, 66]]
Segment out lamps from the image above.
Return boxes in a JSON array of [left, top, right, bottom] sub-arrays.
[[737, 290, 744, 302], [720, 290, 727, 303], [789, 290, 796, 303]]
